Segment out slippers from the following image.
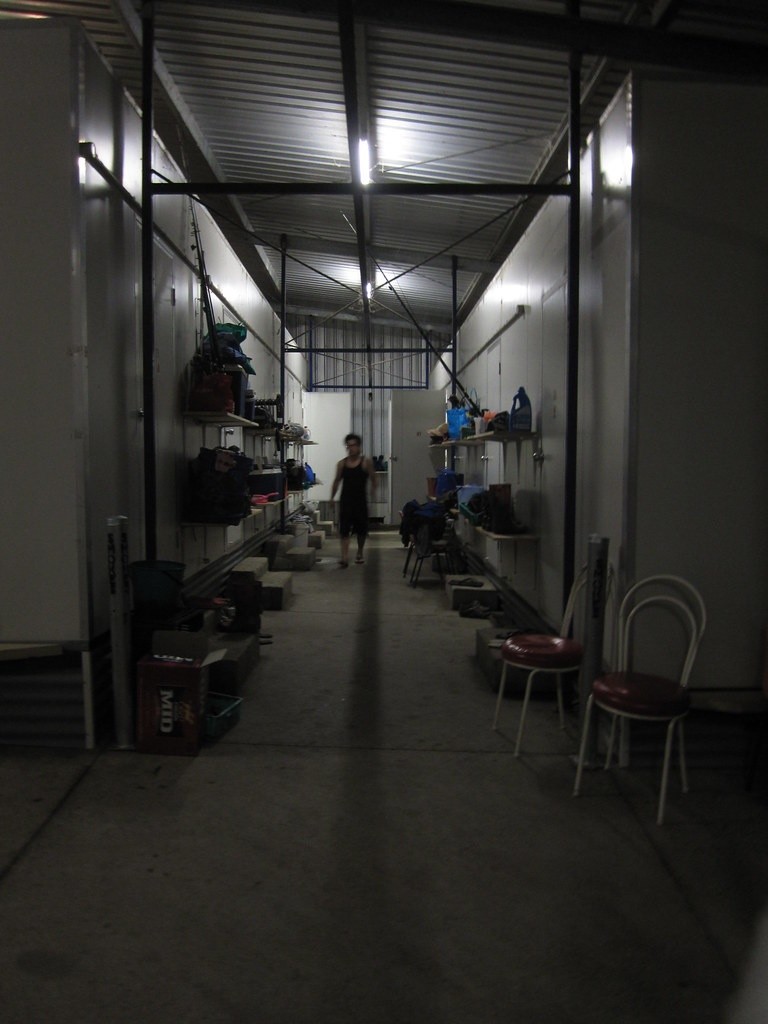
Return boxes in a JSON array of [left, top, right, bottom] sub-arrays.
[[356, 555, 364, 563], [339, 560, 348, 566]]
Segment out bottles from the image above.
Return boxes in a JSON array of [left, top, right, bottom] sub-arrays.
[[508, 386, 532, 431]]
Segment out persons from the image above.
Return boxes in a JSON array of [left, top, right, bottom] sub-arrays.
[[327, 433, 381, 568]]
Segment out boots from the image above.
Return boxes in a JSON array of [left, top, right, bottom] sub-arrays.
[[483, 491, 520, 528], [489, 485, 528, 532]]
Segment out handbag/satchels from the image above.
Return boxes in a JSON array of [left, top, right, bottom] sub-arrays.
[[189, 372, 236, 411], [189, 447, 253, 525]]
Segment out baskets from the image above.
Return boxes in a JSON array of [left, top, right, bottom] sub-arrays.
[[203, 690, 241, 739]]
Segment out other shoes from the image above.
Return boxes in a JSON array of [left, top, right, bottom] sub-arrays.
[[459, 601, 491, 617]]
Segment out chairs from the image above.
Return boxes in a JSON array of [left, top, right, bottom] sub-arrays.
[[491, 560, 613, 756], [571, 575, 706, 825]]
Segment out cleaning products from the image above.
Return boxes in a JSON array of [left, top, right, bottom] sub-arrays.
[[508, 386, 533, 432]]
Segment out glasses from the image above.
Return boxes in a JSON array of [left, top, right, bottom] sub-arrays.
[[346, 444, 358, 449]]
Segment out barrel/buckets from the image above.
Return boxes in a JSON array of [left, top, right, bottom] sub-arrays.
[[435, 467, 465, 507], [130, 559, 186, 619], [456, 483, 484, 519]]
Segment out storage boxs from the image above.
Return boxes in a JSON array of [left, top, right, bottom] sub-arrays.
[[127, 561, 186, 614], [208, 692, 243, 737], [244, 396, 256, 419], [247, 472, 287, 501], [187, 362, 248, 413], [137, 654, 212, 757]]
